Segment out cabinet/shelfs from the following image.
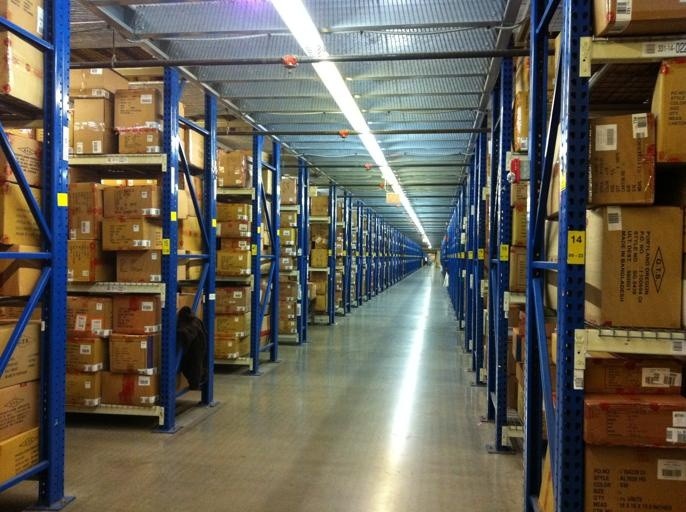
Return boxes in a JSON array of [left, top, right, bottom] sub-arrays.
[[395, 185, 470, 332]]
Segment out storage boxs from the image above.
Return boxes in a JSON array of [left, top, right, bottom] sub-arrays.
[[3, 0, 49, 486], [70, 64, 205, 410], [215, 148, 392, 358], [473, 0, 686, 512]]
